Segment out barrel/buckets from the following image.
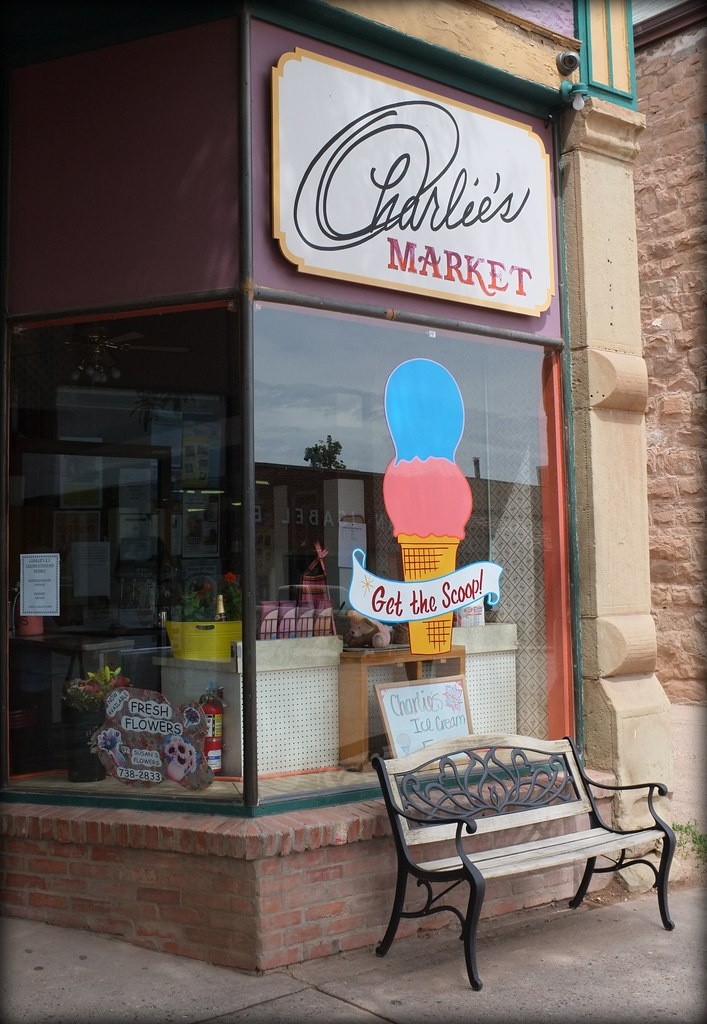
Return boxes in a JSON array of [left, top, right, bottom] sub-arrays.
[[165, 618, 241, 662], [15, 602, 44, 635]]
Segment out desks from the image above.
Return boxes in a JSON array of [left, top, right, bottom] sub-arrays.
[[11, 632, 135, 724], [338, 644, 469, 774]]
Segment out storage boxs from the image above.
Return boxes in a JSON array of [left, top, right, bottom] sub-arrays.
[[279, 599, 298, 639], [258, 600, 279, 639], [298, 599, 315, 637], [314, 598, 335, 635]]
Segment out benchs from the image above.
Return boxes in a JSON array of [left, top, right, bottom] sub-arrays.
[[368, 733, 684, 983]]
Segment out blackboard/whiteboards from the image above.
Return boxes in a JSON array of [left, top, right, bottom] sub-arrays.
[[275, 53, 555, 322], [374, 673, 474, 771]]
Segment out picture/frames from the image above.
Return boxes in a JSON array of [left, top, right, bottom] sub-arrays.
[[58, 436, 104, 510], [178, 492, 221, 559], [52, 510, 103, 553]]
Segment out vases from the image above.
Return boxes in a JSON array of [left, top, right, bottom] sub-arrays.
[[161, 620, 242, 660], [59, 705, 112, 782]]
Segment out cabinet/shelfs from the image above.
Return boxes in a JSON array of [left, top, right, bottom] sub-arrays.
[[323, 477, 370, 614]]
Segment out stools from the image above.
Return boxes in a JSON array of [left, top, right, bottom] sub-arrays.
[[11, 702, 44, 751]]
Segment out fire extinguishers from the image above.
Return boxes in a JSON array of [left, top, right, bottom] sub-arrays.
[[200, 687, 226, 773]]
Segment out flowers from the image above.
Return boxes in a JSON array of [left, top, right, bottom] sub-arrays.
[[173, 569, 243, 621], [63, 660, 136, 709]]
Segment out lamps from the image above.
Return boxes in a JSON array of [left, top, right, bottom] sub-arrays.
[[63, 324, 129, 384], [560, 80, 589, 111]]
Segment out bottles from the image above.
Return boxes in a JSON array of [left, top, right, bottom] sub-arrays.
[[214, 594, 228, 622]]
